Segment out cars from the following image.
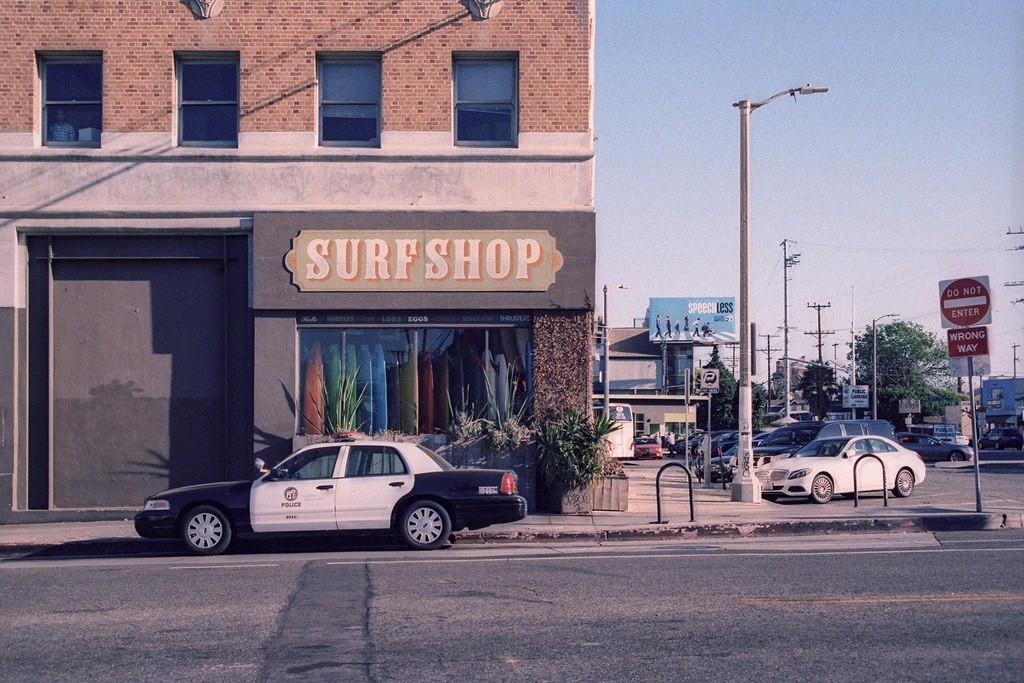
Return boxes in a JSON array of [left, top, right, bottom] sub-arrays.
[[635, 437, 664, 459], [895, 433, 975, 463], [132, 431, 529, 553], [673, 430, 770, 483], [754, 435, 927, 505]]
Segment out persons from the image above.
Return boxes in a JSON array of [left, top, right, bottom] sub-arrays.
[[666, 432, 676, 458], [241, 458, 268, 481], [654, 433, 658, 439]]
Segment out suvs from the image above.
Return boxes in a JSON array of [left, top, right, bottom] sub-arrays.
[[753, 419, 896, 465], [978, 427, 1024, 451]]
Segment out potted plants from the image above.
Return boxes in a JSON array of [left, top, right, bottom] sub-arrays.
[[539, 405, 624, 516]]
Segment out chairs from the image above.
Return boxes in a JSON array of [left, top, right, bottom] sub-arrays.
[[825, 444, 840, 457]]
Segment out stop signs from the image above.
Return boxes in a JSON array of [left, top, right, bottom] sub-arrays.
[[940, 275, 994, 327], [948, 327, 988, 360]]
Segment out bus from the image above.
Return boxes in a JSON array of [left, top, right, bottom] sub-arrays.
[[588, 403, 635, 460]]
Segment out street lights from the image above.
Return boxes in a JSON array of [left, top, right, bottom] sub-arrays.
[[873, 313, 899, 420], [731, 82, 827, 505]]
